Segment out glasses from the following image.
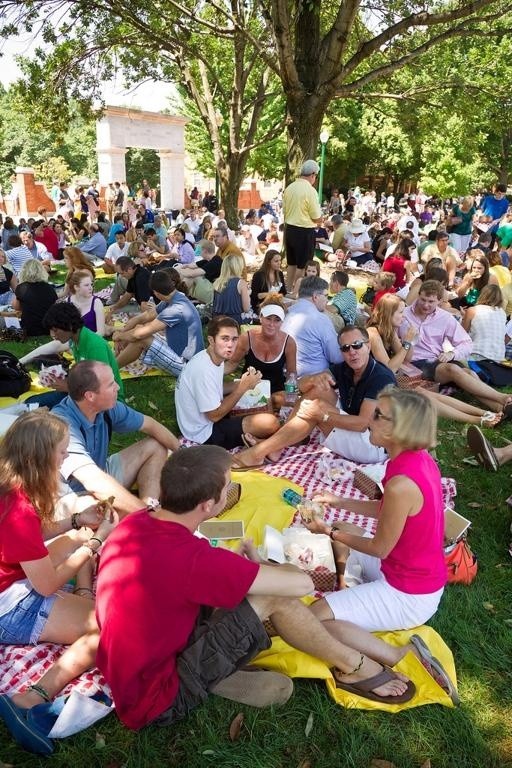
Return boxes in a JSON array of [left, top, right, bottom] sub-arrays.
[[339, 339, 369, 353], [372, 407, 393, 422]]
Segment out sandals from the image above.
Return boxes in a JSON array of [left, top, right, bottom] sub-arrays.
[[480, 411, 508, 429]]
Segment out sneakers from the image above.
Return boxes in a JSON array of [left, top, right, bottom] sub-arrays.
[[215, 480, 242, 519], [465, 423, 500, 473], [335, 248, 352, 271]]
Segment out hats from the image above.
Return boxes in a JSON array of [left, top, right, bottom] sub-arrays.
[[259, 304, 286, 323]]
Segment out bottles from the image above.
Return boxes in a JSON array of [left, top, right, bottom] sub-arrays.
[[280, 486, 305, 507], [282, 368, 297, 403], [148, 296, 155, 304]]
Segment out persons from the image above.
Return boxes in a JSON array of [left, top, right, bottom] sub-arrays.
[[0, 158, 511, 755]]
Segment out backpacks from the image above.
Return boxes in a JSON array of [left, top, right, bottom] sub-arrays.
[[0, 349, 34, 399]]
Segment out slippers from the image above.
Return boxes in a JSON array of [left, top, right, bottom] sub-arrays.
[[207, 669, 295, 710], [331, 661, 416, 705], [0, 692, 55, 755], [230, 453, 266, 473], [241, 432, 275, 464], [408, 631, 461, 707]]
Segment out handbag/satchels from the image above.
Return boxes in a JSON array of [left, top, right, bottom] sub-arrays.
[[443, 535, 478, 587]]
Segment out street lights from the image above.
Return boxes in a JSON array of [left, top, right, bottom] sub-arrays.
[[315, 125, 333, 209], [209, 108, 224, 210]]
